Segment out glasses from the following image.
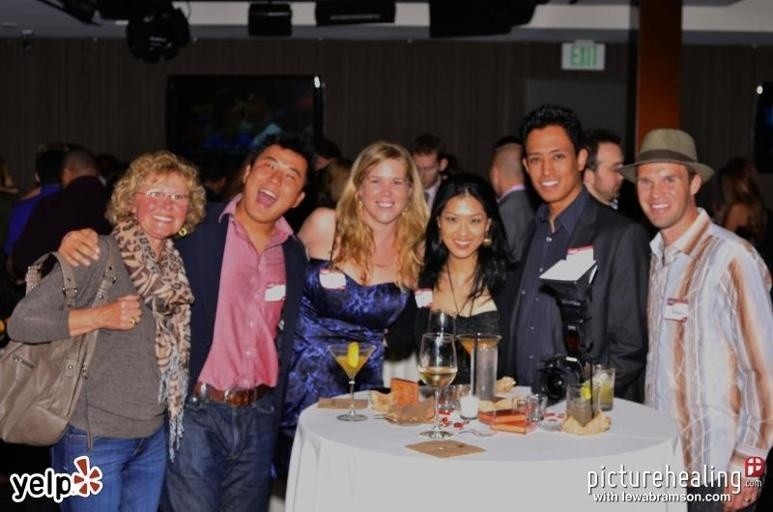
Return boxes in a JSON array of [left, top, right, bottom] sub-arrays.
[[135, 189, 189, 203]]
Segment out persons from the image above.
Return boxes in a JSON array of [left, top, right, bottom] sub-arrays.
[[492, 103, 652, 405], [4, 145, 212, 511], [54, 125, 311, 511], [380, 171, 521, 392], [608, 124, 771, 511], [260, 141, 429, 486]]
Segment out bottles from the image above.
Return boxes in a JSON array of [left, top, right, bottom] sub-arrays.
[[512, 394, 549, 421]]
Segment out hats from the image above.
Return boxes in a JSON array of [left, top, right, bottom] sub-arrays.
[[615, 128, 715, 185]]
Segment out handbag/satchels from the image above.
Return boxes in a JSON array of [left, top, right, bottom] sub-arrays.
[[0, 235, 117, 446]]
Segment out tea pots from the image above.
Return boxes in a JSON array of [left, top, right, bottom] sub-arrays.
[[532, 351, 584, 404]]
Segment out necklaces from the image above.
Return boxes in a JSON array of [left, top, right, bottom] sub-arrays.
[[438, 257, 483, 319]]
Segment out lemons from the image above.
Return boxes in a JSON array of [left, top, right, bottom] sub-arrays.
[[347, 342, 358, 368]]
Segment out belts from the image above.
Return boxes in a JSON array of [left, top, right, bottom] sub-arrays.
[[193, 381, 271, 405]]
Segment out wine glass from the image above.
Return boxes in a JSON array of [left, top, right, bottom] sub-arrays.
[[418, 333, 458, 440], [456, 331, 501, 397], [327, 342, 376, 423]]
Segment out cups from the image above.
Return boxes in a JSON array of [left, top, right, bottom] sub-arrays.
[[566, 384, 599, 435], [468, 349, 497, 407], [458, 395, 478, 420], [590, 367, 614, 412]]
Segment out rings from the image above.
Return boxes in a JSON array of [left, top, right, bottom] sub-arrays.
[[742, 495, 751, 503], [129, 315, 137, 326]]
